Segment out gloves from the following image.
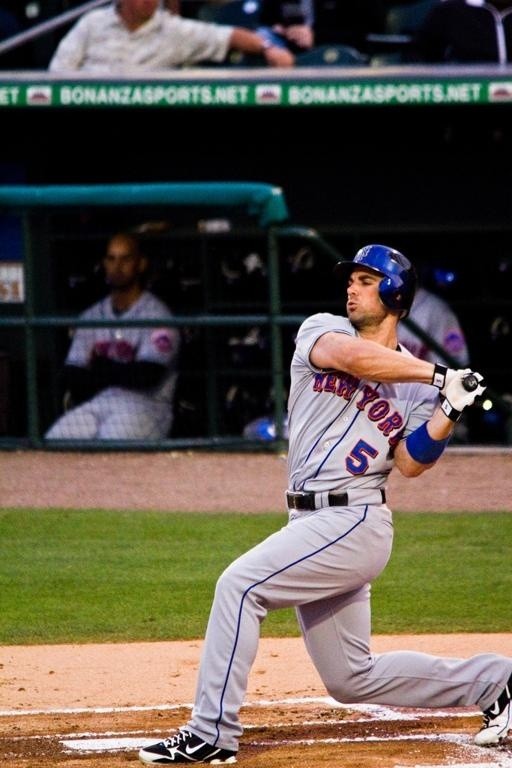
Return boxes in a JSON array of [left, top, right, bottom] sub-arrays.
[[431, 363, 487, 422]]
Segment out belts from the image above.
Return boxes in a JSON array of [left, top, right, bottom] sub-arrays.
[[287, 489, 386, 509]]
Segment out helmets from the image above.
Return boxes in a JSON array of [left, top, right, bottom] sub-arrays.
[[336, 244, 416, 319]]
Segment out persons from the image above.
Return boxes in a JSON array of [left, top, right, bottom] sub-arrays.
[[137, 244, 511, 765]]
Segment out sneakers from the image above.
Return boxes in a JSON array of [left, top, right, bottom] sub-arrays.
[[474, 672, 512, 745], [139, 730, 237, 764]]
